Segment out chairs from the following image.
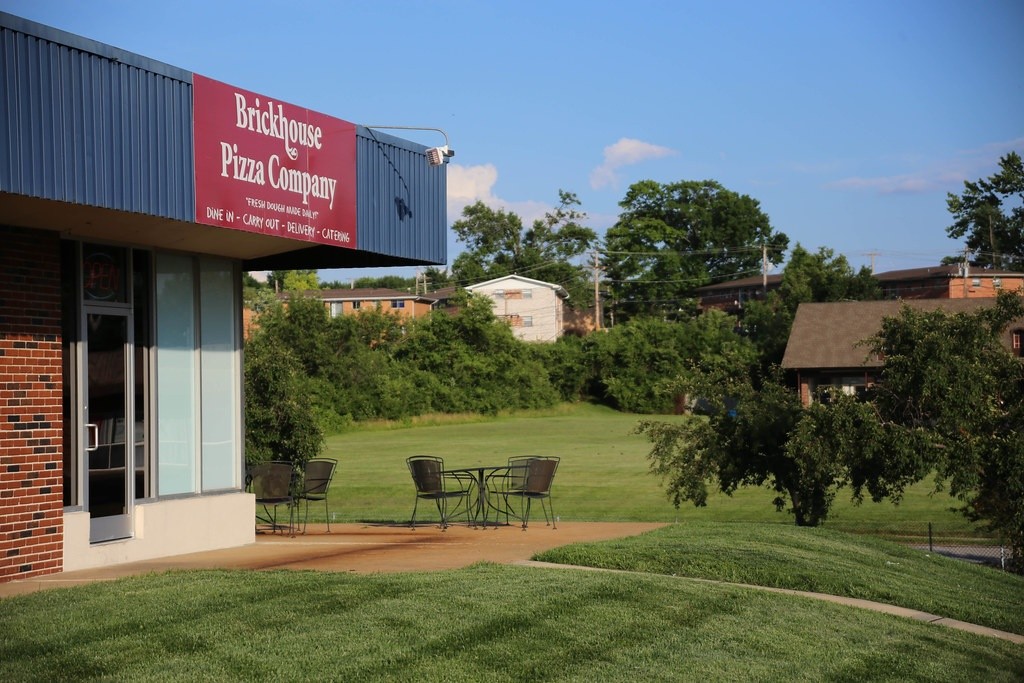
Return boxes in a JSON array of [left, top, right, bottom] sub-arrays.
[[405, 453, 562, 531], [250, 457, 339, 537]]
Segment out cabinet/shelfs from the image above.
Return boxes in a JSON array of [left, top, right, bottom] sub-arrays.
[[90, 415, 144, 474]]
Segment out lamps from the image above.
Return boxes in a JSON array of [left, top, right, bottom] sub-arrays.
[[369, 125, 451, 166]]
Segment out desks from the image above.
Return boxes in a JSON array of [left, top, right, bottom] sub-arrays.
[[427, 466, 532, 529]]
